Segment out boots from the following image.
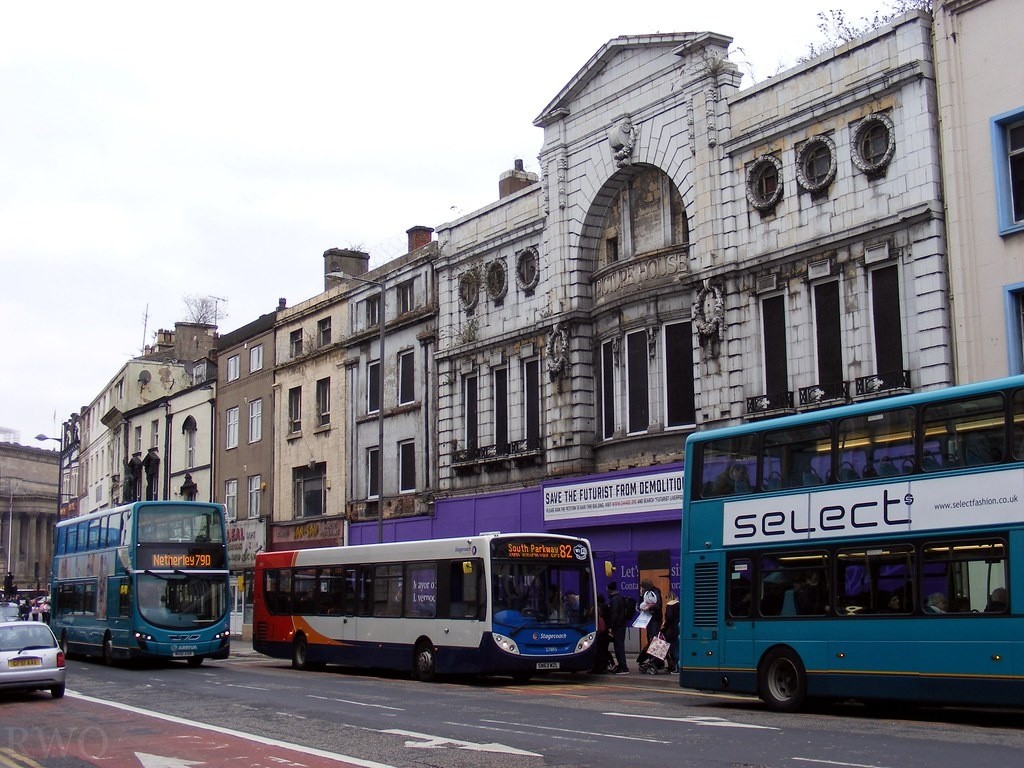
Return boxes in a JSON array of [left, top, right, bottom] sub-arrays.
[[665, 655, 678, 673]]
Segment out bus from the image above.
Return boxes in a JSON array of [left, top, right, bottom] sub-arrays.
[[679, 374, 1024, 713], [50, 501, 230, 668], [253, 531, 616, 683], [0, 589, 47, 613]]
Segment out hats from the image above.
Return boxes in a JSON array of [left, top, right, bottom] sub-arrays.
[[607, 581, 617, 592]]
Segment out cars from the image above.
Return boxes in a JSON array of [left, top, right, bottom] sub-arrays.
[[0, 602, 66, 698]]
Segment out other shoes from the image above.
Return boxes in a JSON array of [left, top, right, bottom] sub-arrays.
[[611, 667, 629, 674], [591, 669, 608, 675]]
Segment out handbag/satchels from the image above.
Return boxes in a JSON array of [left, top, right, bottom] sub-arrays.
[[631, 611, 652, 629], [646, 632, 670, 660], [640, 591, 657, 610]]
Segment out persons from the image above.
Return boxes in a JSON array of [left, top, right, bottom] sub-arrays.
[[712, 463, 751, 497], [18, 598, 50, 625], [547, 579, 679, 675], [876, 591, 908, 613], [985, 587, 1007, 614], [0, 595, 20, 606], [7, 572, 15, 588], [988, 447, 1001, 463], [732, 570, 830, 615], [924, 593, 950, 614]]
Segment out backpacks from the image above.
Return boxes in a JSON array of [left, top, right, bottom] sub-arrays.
[[611, 595, 637, 622], [598, 617, 604, 634]]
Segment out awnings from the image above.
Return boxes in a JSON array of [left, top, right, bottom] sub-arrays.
[[37, 596, 51, 604]]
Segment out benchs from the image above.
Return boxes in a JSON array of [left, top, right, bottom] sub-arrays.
[[780, 589, 801, 616]]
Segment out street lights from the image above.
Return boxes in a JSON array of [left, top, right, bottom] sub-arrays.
[[35, 434, 62, 524], [326, 272, 385, 545]]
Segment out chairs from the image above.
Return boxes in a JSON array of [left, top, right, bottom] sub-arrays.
[[18, 631, 35, 646], [701, 438, 1024, 498]]
